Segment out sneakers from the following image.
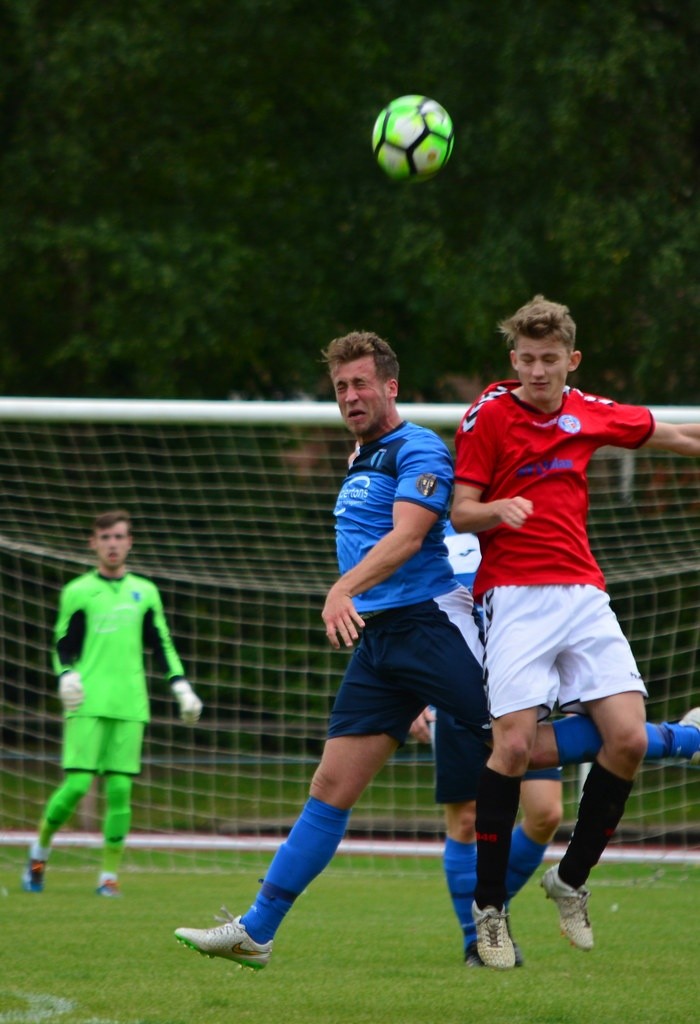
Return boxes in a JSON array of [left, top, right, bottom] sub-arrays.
[[463, 939, 526, 969], [173, 916, 274, 973], [94, 878, 123, 899], [19, 857, 49, 894], [471, 899, 516, 970], [538, 862, 595, 952]]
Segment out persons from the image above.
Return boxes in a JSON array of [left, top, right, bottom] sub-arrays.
[[450, 294, 699, 967], [20, 506, 205, 897], [170, 326, 699, 972], [399, 430, 572, 969]]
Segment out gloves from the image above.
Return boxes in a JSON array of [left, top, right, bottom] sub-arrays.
[[171, 680, 202, 726], [56, 670, 85, 713]]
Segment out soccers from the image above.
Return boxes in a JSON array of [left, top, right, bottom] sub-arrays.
[[369, 92, 458, 186]]
